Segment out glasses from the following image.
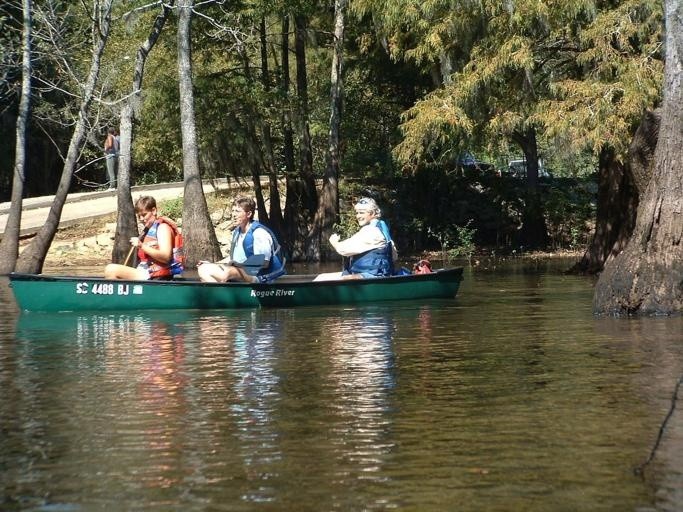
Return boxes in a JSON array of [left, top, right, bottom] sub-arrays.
[[356, 199, 367, 204]]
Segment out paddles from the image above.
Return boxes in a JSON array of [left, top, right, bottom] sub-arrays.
[[200, 254, 265, 275]]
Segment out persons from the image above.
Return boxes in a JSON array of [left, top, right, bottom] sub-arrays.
[[314, 197, 396, 281], [195, 197, 285, 283], [104, 127, 118, 192], [104, 196, 189, 280], [114, 128, 121, 169]]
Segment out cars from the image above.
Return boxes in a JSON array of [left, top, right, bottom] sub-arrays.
[[506, 158, 550, 180]]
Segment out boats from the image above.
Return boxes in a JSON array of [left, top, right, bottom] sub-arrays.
[[7, 266, 463, 315]]
